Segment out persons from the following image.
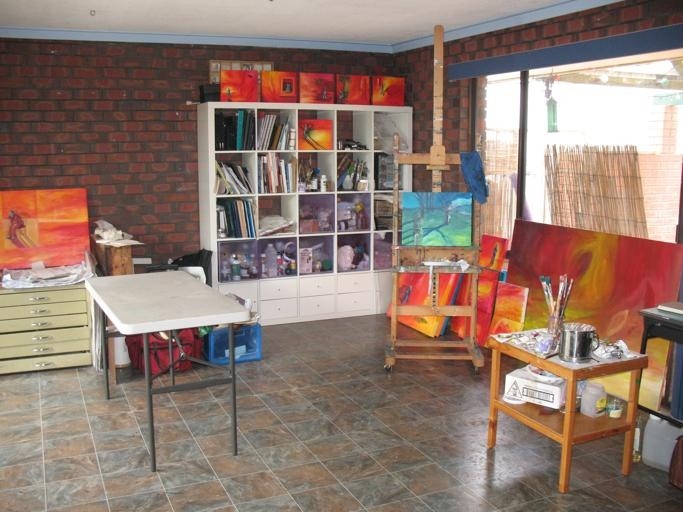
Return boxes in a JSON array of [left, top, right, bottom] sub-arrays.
[[6, 210, 25, 240]]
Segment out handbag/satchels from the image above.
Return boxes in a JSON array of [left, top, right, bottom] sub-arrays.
[[125, 329, 203, 374]]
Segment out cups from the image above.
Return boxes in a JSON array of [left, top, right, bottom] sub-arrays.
[[298, 181, 305, 192], [547, 315, 564, 351]]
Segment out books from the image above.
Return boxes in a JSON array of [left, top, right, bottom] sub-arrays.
[[258, 114, 296, 151], [258, 152, 296, 193], [217, 198, 256, 238], [215, 109, 255, 151], [657, 301, 683, 315], [213, 160, 254, 195]]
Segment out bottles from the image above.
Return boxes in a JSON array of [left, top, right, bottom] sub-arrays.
[[220, 252, 256, 282], [605, 398, 623, 419], [266, 244, 277, 278], [578, 382, 607, 416], [320, 174, 328, 192], [260, 252, 266, 277], [632, 416, 643, 464], [290, 259, 296, 270], [310, 173, 318, 192], [218, 228, 223, 240]]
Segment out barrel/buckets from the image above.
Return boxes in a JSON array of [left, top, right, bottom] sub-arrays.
[[642, 414, 683, 472]]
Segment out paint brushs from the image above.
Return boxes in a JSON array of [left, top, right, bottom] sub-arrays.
[[543, 351, 560, 360], [539, 273, 574, 353]]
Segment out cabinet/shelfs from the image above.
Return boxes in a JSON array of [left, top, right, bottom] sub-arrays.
[[0, 284, 92, 374], [197, 101, 414, 327]]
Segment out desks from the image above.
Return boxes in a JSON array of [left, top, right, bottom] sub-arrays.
[[87, 271, 250, 474], [638, 307, 683, 425], [487, 331, 649, 494]]
